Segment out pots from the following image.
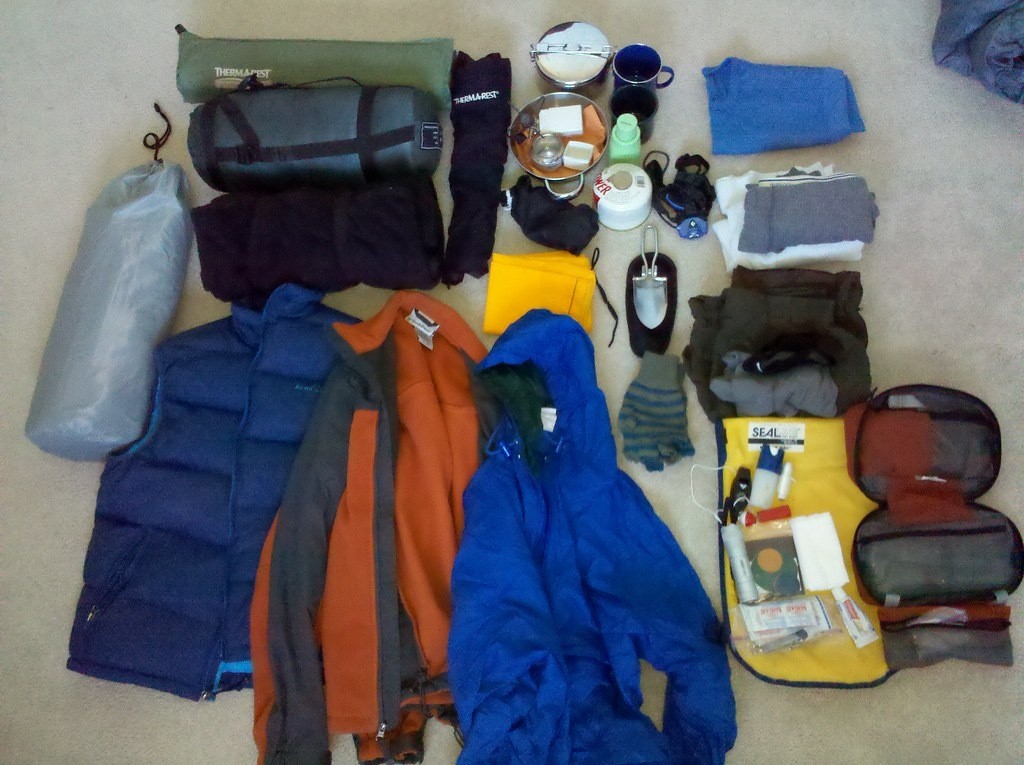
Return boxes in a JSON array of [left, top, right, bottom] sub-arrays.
[[508, 91, 609, 199], [530, 22, 611, 99]]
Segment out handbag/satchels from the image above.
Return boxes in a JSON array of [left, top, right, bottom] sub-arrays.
[[175, 25, 454, 304], [24, 103, 190, 463]]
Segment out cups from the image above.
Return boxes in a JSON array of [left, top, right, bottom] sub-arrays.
[[608, 85, 659, 145], [613, 44, 675, 93]]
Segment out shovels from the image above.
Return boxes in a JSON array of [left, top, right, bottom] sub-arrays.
[[632, 224, 668, 330]]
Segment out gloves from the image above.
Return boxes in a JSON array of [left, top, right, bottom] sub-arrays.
[[616, 350, 695, 473]]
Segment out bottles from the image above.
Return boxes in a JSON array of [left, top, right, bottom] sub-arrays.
[[608, 114, 641, 167]]
